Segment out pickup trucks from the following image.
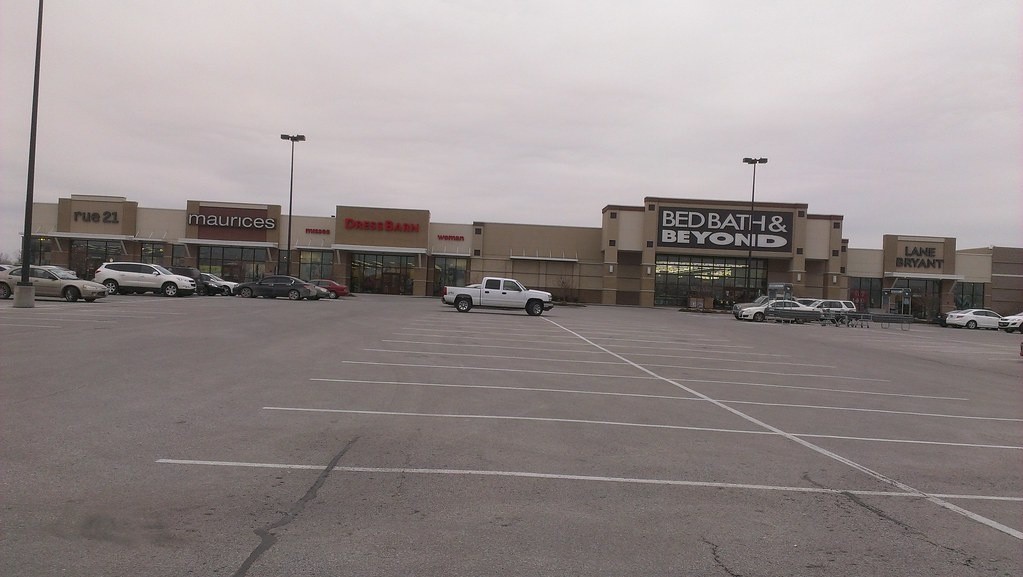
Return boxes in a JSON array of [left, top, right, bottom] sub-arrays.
[[437, 277, 554, 317]]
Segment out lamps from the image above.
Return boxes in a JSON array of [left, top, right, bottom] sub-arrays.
[[646, 266, 651, 275], [796, 273, 802, 282], [832, 276, 837, 284], [609, 265, 614, 272]]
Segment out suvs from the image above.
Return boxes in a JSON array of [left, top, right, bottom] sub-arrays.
[[811, 300, 857, 313], [93, 261, 196, 296], [163, 265, 207, 297]]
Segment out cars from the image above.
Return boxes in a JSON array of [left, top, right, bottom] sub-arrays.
[[60, 266, 76, 276], [998, 310, 1023, 333], [0, 265, 13, 272], [0, 266, 106, 302], [198, 273, 240, 297], [733, 296, 799, 325], [936, 309, 958, 327], [229, 274, 317, 300], [311, 285, 330, 298], [305, 279, 349, 299], [738, 302, 822, 324], [945, 308, 1005, 331], [464, 284, 483, 290]]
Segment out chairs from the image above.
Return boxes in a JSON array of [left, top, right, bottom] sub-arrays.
[[820, 312, 873, 330]]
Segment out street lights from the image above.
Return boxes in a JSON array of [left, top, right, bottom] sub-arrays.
[[280, 133, 308, 276], [742, 156, 769, 303]]
